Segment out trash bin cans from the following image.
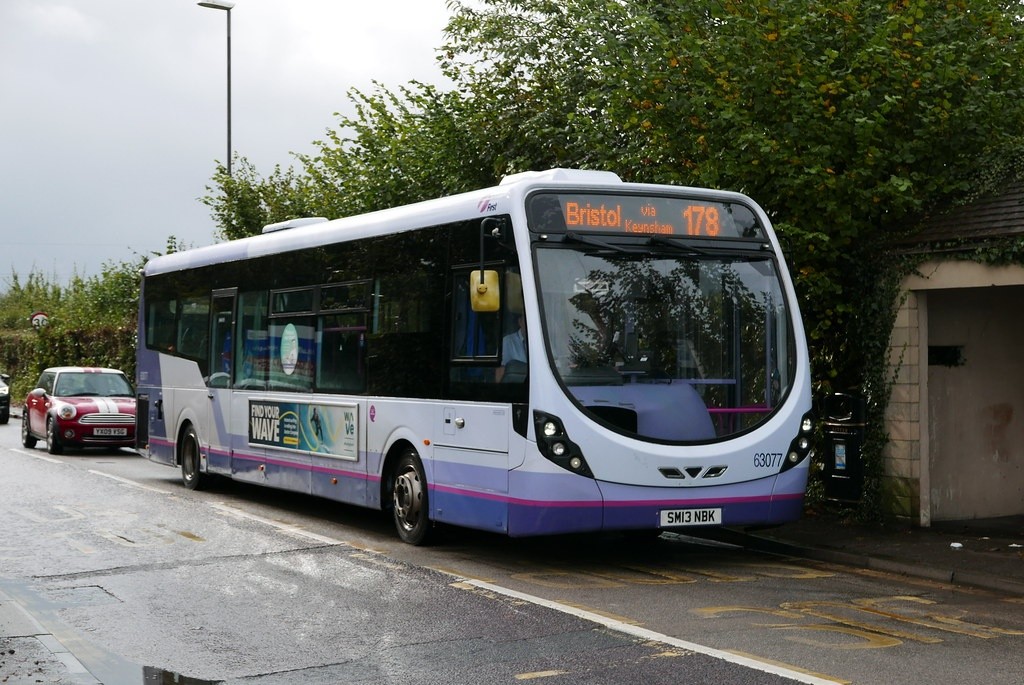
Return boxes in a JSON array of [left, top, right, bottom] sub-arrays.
[[821, 393, 869, 511]]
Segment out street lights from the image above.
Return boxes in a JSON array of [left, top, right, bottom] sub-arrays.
[[195, 0, 236, 177]]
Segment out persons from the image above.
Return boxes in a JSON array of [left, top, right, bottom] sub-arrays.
[[496, 305, 562, 385]]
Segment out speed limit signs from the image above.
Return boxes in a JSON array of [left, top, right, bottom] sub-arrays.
[[30, 312, 50, 332]]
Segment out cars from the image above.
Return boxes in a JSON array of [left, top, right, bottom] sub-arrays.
[[0, 373, 10, 425], [22, 367, 135, 455]]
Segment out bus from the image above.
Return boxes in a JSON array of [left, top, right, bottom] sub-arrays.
[[135, 167, 813, 547]]
